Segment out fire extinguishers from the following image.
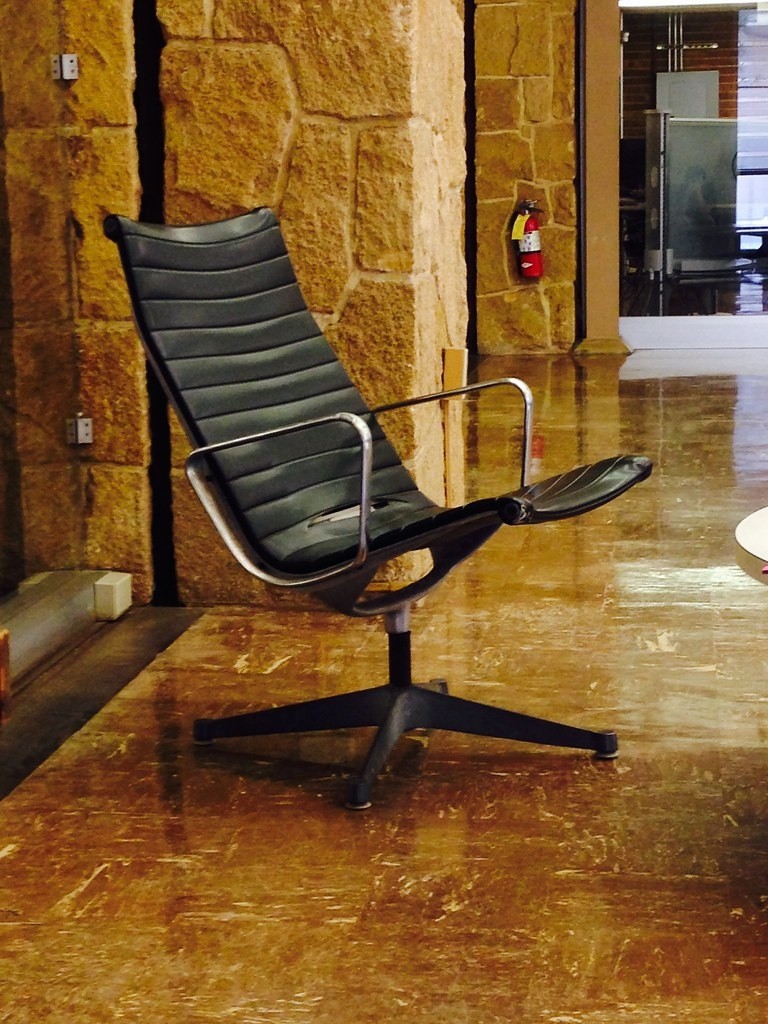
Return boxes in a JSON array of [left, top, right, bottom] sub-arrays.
[[516, 199, 546, 279]]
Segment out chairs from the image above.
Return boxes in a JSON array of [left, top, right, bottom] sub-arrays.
[[101, 200, 653, 812]]
